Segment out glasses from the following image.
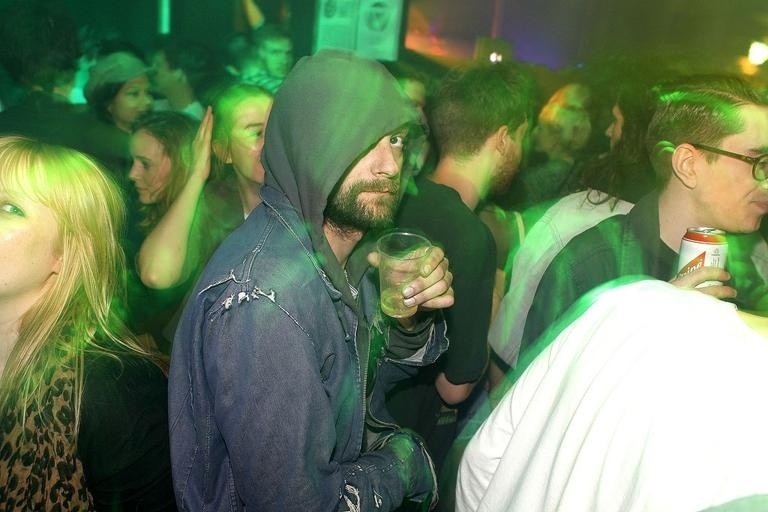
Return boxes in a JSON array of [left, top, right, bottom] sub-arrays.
[[691, 141, 768, 183]]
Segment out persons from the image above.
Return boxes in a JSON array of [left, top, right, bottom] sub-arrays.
[[0, 24, 291, 511], [384, 58, 767, 512], [169, 47, 455, 511]]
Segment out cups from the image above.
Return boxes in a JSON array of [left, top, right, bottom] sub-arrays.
[[376, 233, 431, 320]]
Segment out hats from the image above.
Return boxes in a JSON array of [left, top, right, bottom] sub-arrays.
[[83, 52, 157, 100]]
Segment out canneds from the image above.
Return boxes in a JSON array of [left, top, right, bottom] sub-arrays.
[[676, 225, 728, 290]]
